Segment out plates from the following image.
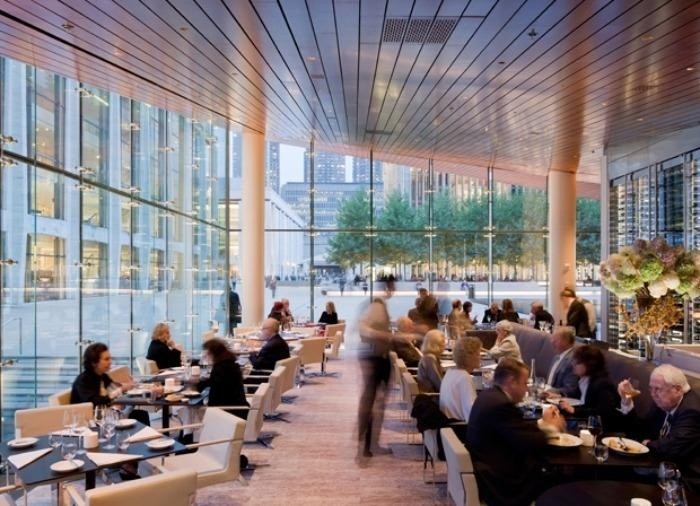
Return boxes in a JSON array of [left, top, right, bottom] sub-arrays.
[[116, 418, 137, 428], [50, 459, 84, 472], [547, 397, 582, 406], [548, 432, 583, 448], [7, 437, 38, 448], [145, 438, 175, 450], [181, 390, 201, 396], [601, 436, 650, 456]]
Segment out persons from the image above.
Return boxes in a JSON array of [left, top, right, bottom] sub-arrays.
[[146, 323, 183, 368], [356, 272, 422, 461], [221, 287, 241, 336], [583, 274, 592, 284], [319, 302, 338, 325], [242, 318, 291, 392], [71, 343, 149, 479], [268, 299, 294, 322], [174, 338, 251, 466], [117, 271, 519, 287], [394, 286, 700, 506]]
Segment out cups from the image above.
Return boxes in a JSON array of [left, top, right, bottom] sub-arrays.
[[116, 431, 131, 450], [80, 431, 98, 449], [631, 498, 651, 506], [60, 438, 77, 460], [165, 378, 175, 389], [543, 404, 551, 416], [192, 366, 200, 375], [48, 428, 63, 448]]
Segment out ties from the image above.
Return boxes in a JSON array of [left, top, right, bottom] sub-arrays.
[[661, 414, 672, 440], [100, 380, 107, 396]]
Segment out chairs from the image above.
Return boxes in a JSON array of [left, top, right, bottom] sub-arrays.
[[388, 351, 401, 386], [402, 371, 441, 446], [440, 427, 482, 506], [394, 357, 420, 423], [412, 393, 469, 487]]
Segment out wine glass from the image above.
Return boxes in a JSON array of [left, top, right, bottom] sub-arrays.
[[102, 409, 116, 450], [63, 411, 76, 438], [94, 404, 107, 443], [591, 434, 609, 461], [587, 416, 603, 454], [657, 461, 682, 506]]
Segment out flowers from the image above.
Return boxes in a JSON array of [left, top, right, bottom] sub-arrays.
[[596, 236, 700, 304]]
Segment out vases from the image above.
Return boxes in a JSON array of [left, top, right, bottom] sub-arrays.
[[635, 296, 656, 357]]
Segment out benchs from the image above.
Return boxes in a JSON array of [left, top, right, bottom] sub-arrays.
[[509, 321, 700, 478]]
